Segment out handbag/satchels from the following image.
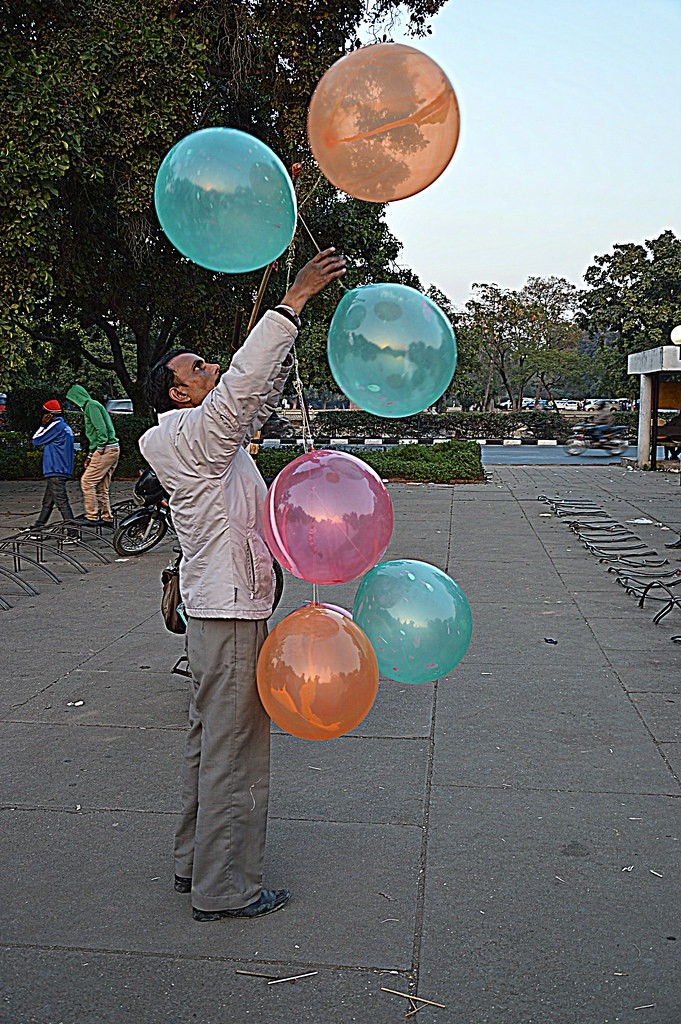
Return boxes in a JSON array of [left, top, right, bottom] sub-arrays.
[[160, 566, 186, 634]]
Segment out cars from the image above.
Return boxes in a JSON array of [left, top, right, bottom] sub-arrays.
[[106, 399, 134, 416], [501, 398, 640, 411]]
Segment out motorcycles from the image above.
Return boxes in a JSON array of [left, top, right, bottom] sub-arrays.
[[112, 467, 274, 556], [565, 414, 629, 454]]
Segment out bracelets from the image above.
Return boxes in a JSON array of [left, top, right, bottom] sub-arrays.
[[274, 305, 302, 326]]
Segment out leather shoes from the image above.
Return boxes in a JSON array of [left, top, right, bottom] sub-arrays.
[[173, 873, 193, 892], [192, 887, 291, 922]]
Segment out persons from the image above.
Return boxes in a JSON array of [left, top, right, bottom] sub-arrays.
[[65, 384, 122, 528], [137, 245, 348, 922], [19, 400, 84, 546], [670, 412, 681, 460]]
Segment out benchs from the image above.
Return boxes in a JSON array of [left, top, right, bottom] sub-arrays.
[[650, 426, 681, 447]]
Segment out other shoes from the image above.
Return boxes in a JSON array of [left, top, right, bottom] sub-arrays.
[[78, 518, 99, 527], [19, 528, 45, 540], [98, 519, 114, 528], [63, 535, 82, 544]]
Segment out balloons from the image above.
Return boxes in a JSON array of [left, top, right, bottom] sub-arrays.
[[155, 127, 297, 274], [256, 600, 379, 742], [328, 283, 458, 417], [307, 44, 460, 203], [262, 449, 394, 583], [352, 557, 473, 685]]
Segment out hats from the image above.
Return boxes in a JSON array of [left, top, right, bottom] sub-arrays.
[[43, 400, 62, 412]]
